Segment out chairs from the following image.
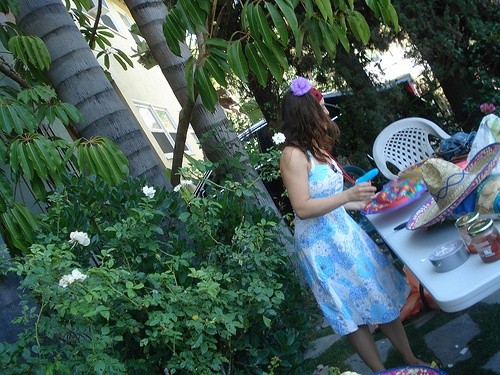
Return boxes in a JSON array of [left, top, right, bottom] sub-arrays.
[[373, 118, 452, 180]]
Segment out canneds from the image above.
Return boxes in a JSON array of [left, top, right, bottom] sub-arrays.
[[456, 211, 500, 264]]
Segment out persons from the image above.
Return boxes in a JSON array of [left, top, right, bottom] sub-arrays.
[[277, 75, 433, 373]]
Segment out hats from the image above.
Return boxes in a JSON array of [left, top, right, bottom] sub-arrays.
[[405, 142, 499, 232]]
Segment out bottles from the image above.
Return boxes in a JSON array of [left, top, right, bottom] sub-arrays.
[[468, 217, 500, 264], [455, 211, 486, 254]]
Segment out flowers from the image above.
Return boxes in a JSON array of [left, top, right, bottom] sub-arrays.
[[290, 77, 312, 96]]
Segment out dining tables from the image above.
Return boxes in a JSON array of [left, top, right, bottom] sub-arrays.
[[365, 163, 499, 313]]
